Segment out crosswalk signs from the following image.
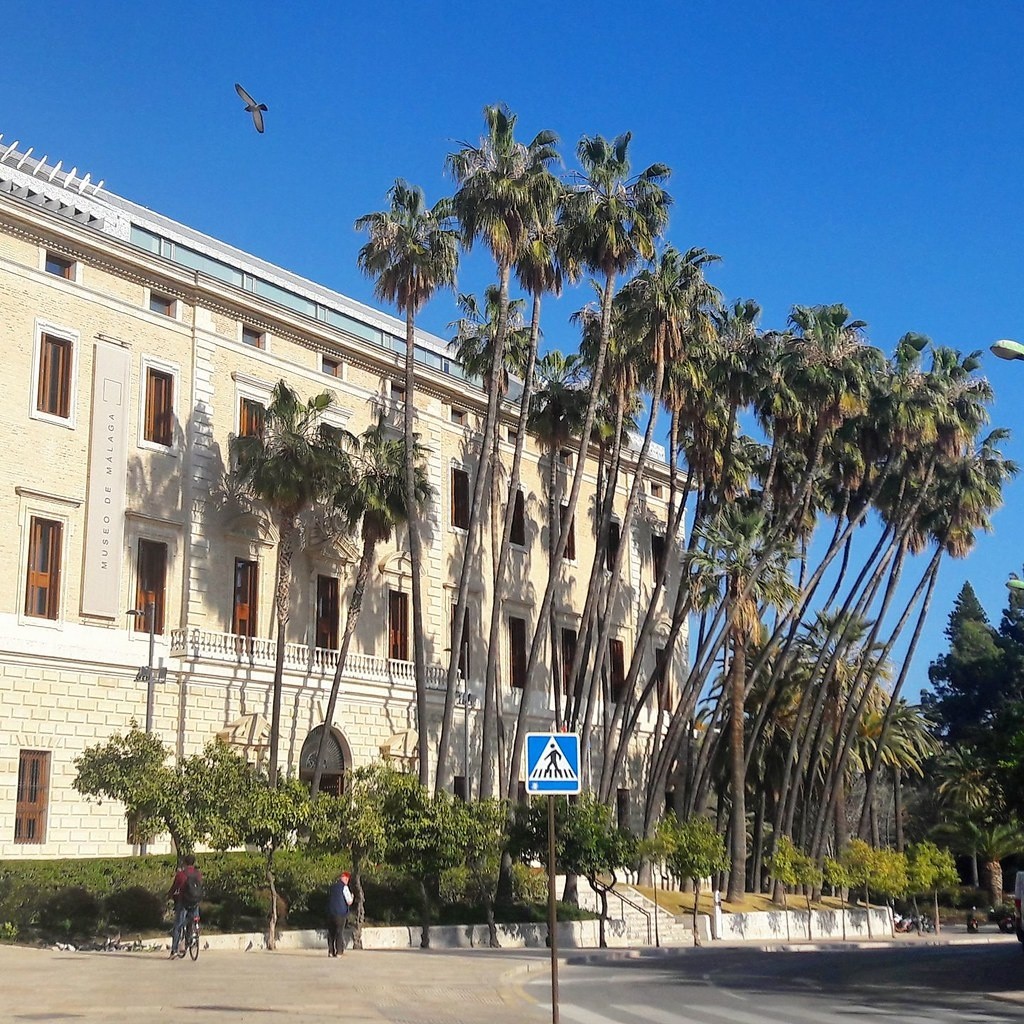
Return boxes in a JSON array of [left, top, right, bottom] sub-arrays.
[[524, 732, 582, 794]]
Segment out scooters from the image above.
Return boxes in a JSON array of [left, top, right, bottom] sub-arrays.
[[896, 906, 1017, 933]]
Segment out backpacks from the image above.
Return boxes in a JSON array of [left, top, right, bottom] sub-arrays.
[[183, 869, 203, 904]]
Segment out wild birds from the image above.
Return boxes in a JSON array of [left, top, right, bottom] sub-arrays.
[[235, 82, 269, 134]]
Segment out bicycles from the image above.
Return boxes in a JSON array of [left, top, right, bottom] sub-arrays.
[[170, 896, 200, 961]]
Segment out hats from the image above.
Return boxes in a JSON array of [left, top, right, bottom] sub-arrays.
[[340, 871, 352, 881]]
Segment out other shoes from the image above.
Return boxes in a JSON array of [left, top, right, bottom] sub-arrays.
[[168, 952, 178, 960], [327, 954, 335, 957], [336, 953, 343, 958]]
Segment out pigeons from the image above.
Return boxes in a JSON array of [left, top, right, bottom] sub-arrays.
[[245, 940, 253, 952], [204, 941, 209, 950], [54, 935, 171, 953]]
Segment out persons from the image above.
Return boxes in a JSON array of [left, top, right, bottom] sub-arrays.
[[969, 906, 976, 919], [167, 854, 202, 960], [327, 872, 354, 958]]
